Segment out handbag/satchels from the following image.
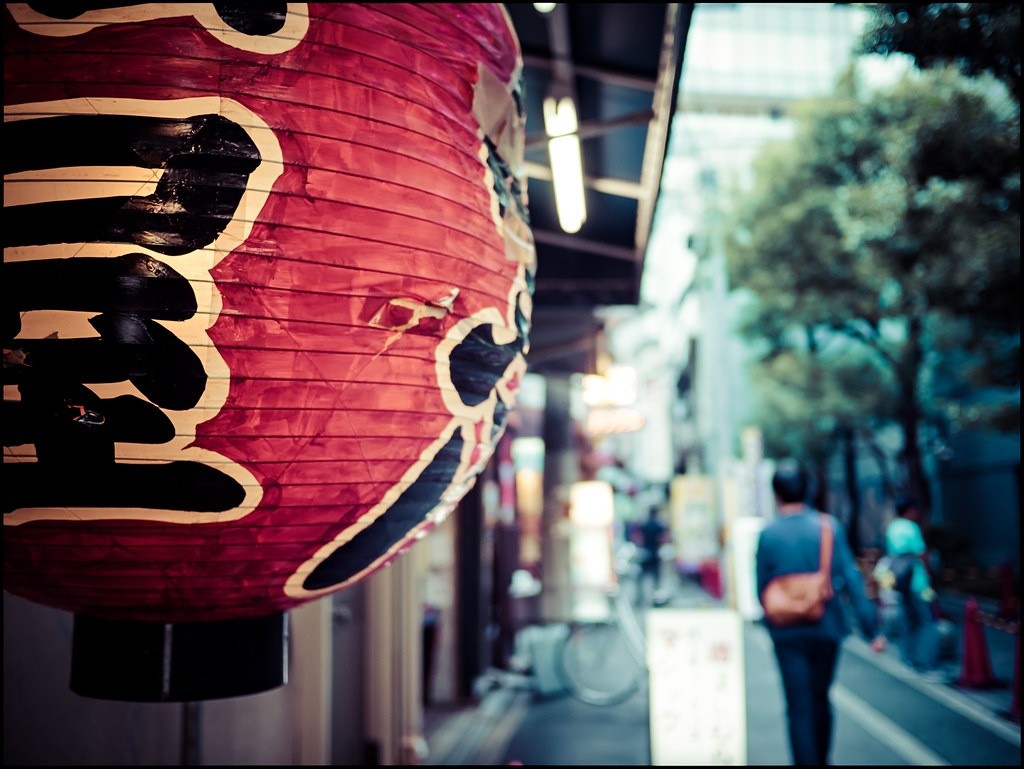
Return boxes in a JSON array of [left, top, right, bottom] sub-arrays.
[[762, 570, 834, 624]]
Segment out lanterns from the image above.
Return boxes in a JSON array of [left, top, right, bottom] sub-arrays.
[[0, 0, 539, 708]]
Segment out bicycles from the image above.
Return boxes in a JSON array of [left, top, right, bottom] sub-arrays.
[[556, 538, 648, 708]]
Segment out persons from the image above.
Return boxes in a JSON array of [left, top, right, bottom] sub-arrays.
[[630, 506, 667, 607], [874, 504, 938, 650], [756, 457, 885, 766]]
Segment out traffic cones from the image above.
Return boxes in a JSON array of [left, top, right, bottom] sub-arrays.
[[945, 601, 1007, 693]]
